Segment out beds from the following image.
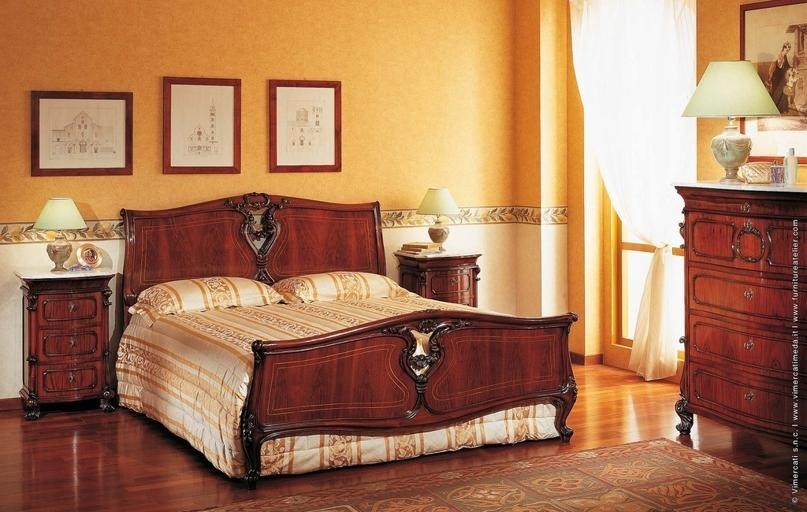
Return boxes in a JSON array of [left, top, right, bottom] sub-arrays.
[[112, 191, 580, 492]]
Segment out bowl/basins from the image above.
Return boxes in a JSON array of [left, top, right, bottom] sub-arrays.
[[738, 163, 775, 185]]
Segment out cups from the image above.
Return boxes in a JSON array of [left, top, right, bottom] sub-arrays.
[[770, 165, 783, 184]]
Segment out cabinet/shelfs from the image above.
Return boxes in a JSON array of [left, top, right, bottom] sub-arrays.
[[669, 181, 806, 453]]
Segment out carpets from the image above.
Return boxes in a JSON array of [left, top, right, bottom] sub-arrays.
[[184, 434, 805, 511]]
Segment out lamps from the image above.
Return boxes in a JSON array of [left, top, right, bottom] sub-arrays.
[[415, 188, 460, 253], [679, 59, 783, 186], [31, 197, 89, 274]]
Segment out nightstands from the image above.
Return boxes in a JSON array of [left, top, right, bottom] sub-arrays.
[[11, 268, 121, 423], [392, 250, 481, 310]]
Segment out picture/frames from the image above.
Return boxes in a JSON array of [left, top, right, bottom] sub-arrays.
[[27, 88, 135, 178], [266, 79, 343, 175], [159, 75, 244, 176], [736, 0, 807, 166]]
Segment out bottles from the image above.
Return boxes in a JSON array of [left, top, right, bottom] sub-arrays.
[[783, 147, 799, 185]]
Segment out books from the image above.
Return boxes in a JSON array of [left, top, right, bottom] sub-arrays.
[[393, 241, 441, 259]]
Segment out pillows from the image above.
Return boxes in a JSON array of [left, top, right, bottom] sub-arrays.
[[127, 274, 285, 328], [270, 269, 420, 304]]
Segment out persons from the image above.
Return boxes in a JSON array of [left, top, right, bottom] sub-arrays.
[[763, 40, 792, 113]]
